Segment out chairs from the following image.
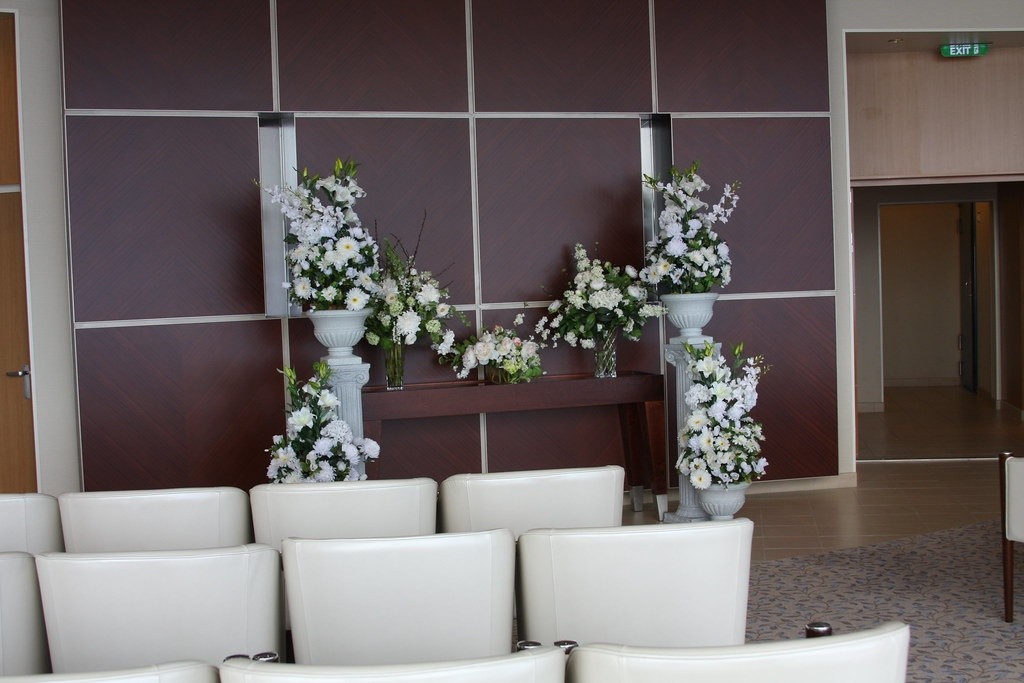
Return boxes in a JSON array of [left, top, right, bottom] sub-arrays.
[[998, 449, 1024, 620], [0, 463, 910, 683]]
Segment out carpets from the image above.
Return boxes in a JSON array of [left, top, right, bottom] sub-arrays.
[[744, 515, 1024, 683]]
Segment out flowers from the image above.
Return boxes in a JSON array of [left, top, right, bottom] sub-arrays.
[[262, 359, 381, 484], [524, 243, 669, 348], [255, 156, 386, 311], [628, 160, 743, 294], [365, 207, 456, 346], [448, 313, 546, 385], [676, 340, 769, 486]]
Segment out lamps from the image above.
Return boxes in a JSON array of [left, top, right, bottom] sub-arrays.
[[637, 112, 679, 490], [256, 110, 303, 440]]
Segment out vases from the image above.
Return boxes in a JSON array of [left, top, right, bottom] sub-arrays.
[[308, 308, 376, 360], [381, 337, 404, 391], [486, 366, 522, 384], [697, 482, 750, 521], [661, 293, 715, 343], [596, 328, 618, 378]]
